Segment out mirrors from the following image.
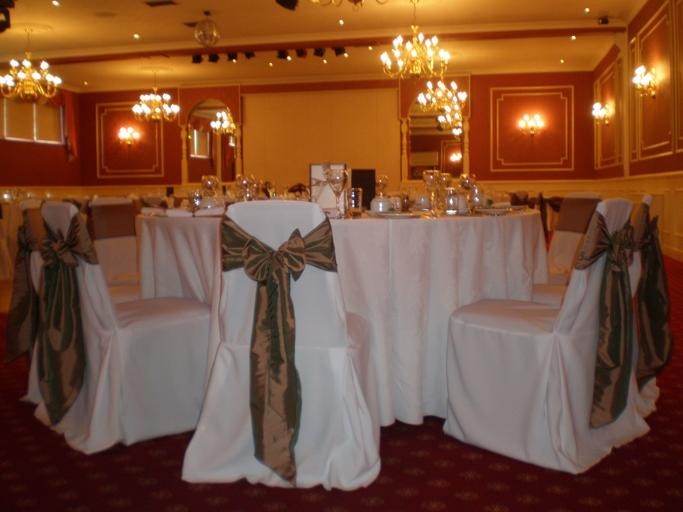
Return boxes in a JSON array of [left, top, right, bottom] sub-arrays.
[[400, 74, 471, 185], [182, 85, 241, 184]]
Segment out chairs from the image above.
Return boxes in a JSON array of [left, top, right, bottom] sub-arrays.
[[34, 202, 216, 451], [181, 199, 384, 494], [23, 208, 48, 248], [547, 196, 602, 283], [0, 200, 24, 281], [531, 194, 663, 417], [442, 200, 651, 477]]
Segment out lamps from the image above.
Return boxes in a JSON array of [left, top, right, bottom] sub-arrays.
[[194, 17, 222, 48], [381, 2, 451, 83], [591, 102, 609, 126], [130, 72, 181, 124], [210, 110, 235, 135], [0, 23, 61, 106], [116, 127, 139, 148], [632, 65, 658, 98], [417, 82, 467, 140], [517, 113, 545, 138]]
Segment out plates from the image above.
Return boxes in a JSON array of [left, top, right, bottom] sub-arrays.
[[474, 207, 510, 215]]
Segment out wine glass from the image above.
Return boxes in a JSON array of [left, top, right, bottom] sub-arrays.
[[188, 168, 480, 218]]
